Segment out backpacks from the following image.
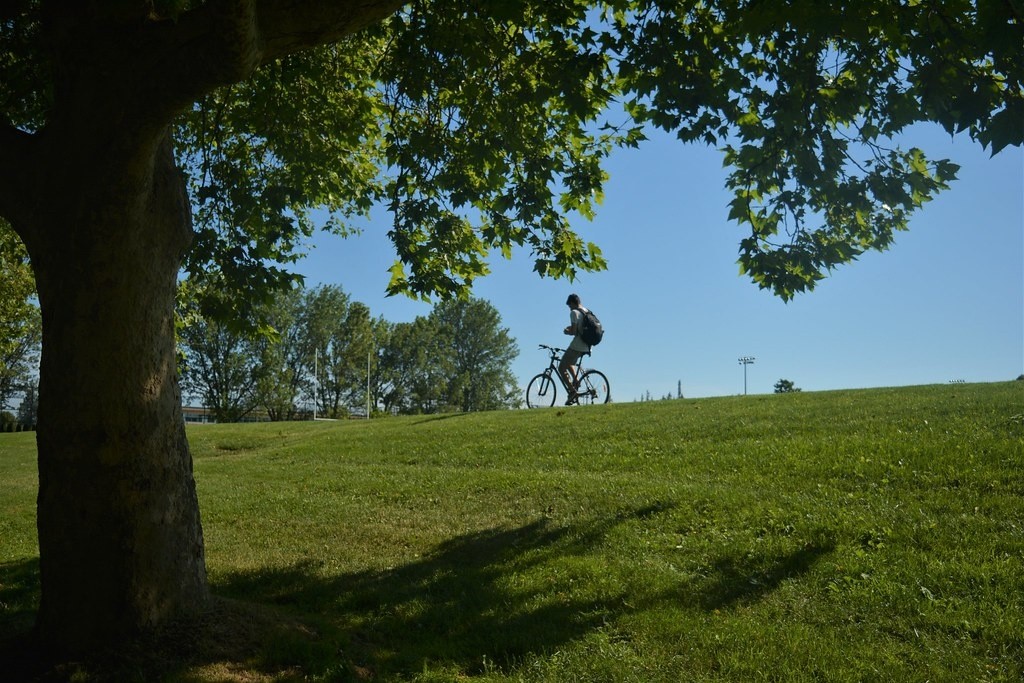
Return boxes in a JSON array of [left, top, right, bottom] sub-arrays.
[[571, 307, 604, 345]]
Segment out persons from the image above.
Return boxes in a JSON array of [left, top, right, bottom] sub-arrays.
[[558, 294, 591, 404]]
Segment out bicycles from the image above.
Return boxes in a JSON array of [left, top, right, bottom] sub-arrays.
[[526, 344, 610, 408]]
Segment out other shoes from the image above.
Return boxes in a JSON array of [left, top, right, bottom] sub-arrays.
[[572, 381, 581, 387], [565, 389, 579, 405]]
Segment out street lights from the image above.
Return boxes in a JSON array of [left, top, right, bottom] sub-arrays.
[[738, 356, 755, 395]]
